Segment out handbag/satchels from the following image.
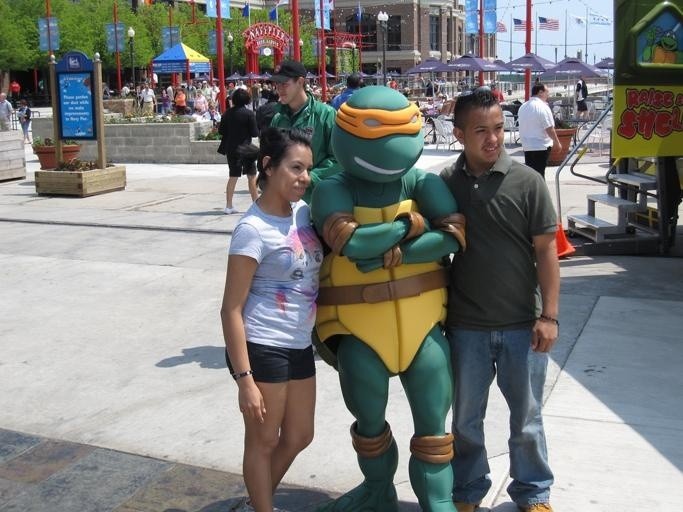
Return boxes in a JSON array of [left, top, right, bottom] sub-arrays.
[[238, 142, 259, 160]]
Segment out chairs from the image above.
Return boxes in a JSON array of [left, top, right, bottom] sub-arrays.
[[419, 89, 612, 156]]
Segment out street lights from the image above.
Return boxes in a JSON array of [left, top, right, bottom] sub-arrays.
[[226, 33, 233, 75], [299, 38, 302, 63], [127, 26, 135, 109], [351, 42, 356, 73], [377, 11, 388, 87]]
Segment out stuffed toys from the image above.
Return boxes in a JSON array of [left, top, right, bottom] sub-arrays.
[[309, 83, 468, 512]]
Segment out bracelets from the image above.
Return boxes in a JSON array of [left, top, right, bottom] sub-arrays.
[[538, 313, 559, 327], [232, 368, 254, 382]]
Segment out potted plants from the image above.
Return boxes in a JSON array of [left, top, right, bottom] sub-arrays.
[[30, 137, 82, 170], [536, 117, 576, 166]]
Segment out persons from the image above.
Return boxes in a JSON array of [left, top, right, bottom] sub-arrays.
[[387, 77, 464, 97], [0, 80, 32, 145], [306, 82, 346, 100], [102, 82, 111, 100], [439, 89, 560, 511], [218, 127, 324, 512], [217, 87, 261, 215], [121, 80, 278, 126], [574, 74, 588, 120]]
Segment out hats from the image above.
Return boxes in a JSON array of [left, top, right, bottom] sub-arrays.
[[268, 59, 306, 84]]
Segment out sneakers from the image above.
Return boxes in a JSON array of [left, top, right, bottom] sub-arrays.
[[452, 499, 481, 512], [518, 502, 552, 512], [224, 207, 237, 214]]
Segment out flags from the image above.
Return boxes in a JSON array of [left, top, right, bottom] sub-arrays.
[[240, 5, 249, 18], [513, 18, 533, 33], [268, 8, 276, 20], [495, 22, 506, 33], [538, 17, 559, 32], [589, 12, 611, 26], [565, 14, 586, 29]]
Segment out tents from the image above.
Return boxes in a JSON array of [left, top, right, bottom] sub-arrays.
[[151, 42, 214, 85]]
